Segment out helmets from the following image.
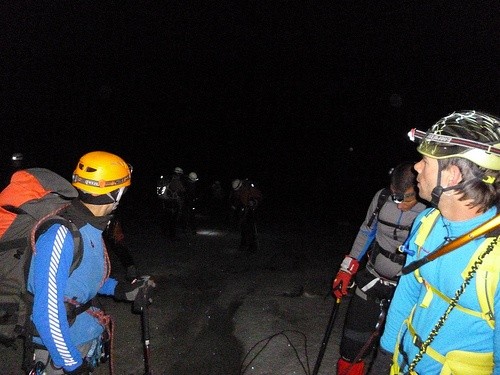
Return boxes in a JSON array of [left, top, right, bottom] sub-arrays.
[[386, 161, 418, 203], [175, 167, 183, 174], [71, 151, 133, 197], [417, 111, 500, 171], [232, 179, 242, 190], [188, 172, 199, 181]]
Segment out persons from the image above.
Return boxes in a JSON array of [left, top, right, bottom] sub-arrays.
[[232, 178, 261, 251], [24, 152, 158, 375], [180, 173, 203, 235], [378, 110, 500, 374], [331, 161, 428, 375], [169, 167, 187, 211], [5, 152, 23, 179], [155, 173, 181, 239]]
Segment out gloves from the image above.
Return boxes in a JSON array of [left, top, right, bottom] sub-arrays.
[[114, 275, 157, 304], [332, 256, 358, 299], [62, 358, 94, 375]]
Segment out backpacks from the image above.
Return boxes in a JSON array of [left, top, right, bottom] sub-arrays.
[[0, 168, 79, 350]]
